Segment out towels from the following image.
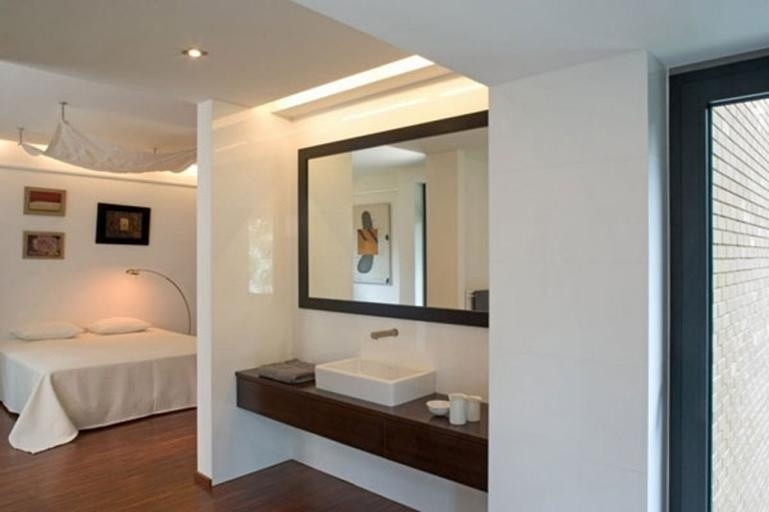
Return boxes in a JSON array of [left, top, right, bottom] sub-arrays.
[[254, 357, 316, 384]]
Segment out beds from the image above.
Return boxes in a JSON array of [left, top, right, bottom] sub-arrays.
[[1, 329, 197, 433]]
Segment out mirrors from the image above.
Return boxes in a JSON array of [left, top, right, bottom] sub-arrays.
[[297, 111, 488, 330]]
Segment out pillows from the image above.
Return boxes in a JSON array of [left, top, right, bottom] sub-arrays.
[[10, 315, 151, 343]]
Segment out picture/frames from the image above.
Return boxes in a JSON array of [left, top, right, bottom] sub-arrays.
[[21, 231, 66, 263], [23, 185, 67, 217], [95, 203, 150, 247]]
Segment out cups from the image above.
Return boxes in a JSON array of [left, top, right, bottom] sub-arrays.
[[465, 397, 484, 423], [447, 391, 467, 426]]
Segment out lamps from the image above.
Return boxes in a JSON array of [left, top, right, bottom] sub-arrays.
[[126, 268, 193, 336]]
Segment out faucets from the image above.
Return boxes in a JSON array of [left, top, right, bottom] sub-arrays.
[[371, 328, 398, 338]]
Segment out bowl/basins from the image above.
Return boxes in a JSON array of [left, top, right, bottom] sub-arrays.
[[425, 399, 451, 417]]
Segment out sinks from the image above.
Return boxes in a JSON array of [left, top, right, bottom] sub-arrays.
[[315, 357, 437, 407]]
